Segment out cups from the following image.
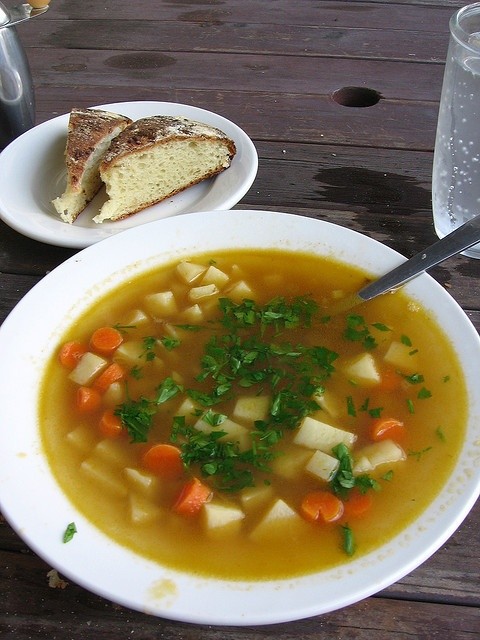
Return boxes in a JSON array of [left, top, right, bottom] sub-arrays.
[[431, 3, 480, 260]]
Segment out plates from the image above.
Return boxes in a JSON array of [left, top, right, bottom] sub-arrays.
[[0, 100, 259, 249]]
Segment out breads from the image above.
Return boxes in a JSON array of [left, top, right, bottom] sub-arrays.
[[91, 116, 237, 224], [51, 106, 133, 224]]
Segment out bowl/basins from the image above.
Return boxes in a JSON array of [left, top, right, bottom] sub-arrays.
[[1, 210, 480, 628]]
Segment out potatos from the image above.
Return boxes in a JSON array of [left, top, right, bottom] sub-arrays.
[[59, 260, 417, 545]]
